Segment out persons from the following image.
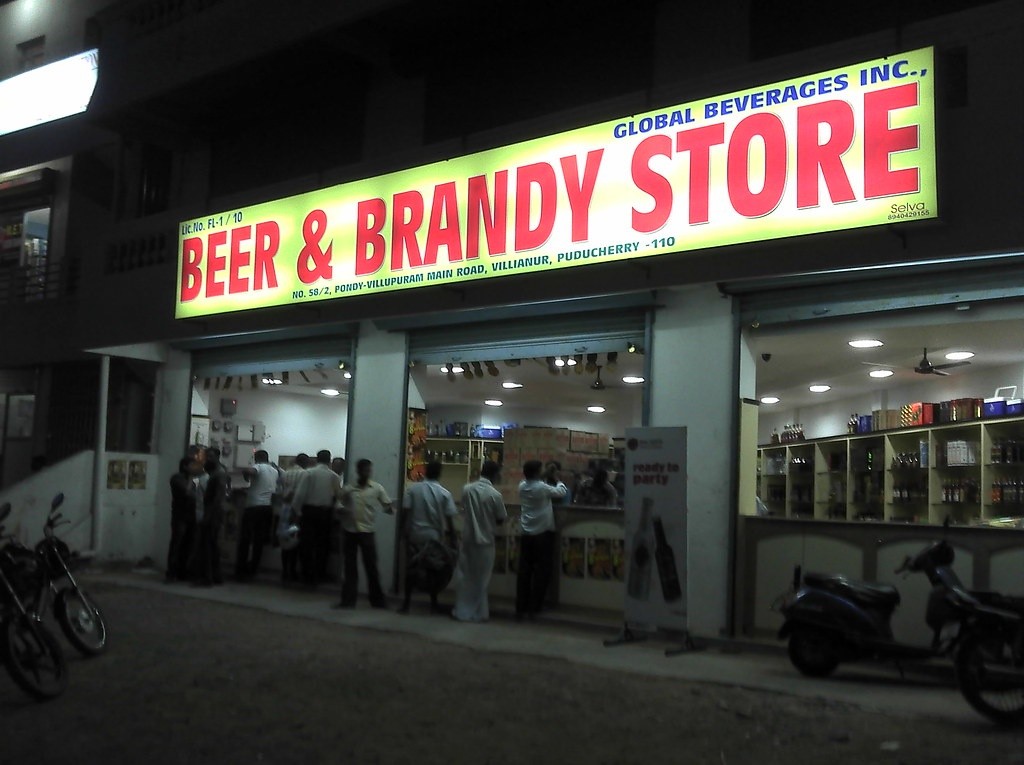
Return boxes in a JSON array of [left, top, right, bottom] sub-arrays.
[[445, 461, 507, 622], [334, 460, 393, 610], [164, 443, 341, 587], [395, 461, 458, 615], [546, 459, 618, 508], [515, 461, 567, 620]]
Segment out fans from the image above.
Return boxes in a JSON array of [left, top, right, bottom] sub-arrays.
[[861, 347, 971, 375], [566, 365, 631, 390]]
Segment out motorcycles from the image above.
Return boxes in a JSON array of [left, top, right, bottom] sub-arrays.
[[25, 494, 111, 657], [0, 502, 74, 699]]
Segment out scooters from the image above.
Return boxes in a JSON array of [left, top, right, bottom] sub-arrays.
[[770, 513, 1024, 724]]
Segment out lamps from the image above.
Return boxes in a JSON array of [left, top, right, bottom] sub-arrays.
[[627, 341, 636, 353]]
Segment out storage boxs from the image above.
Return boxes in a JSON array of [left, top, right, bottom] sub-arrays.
[[857, 385, 1024, 432]]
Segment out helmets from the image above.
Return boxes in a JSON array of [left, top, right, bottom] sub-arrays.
[[278, 525, 300, 549]]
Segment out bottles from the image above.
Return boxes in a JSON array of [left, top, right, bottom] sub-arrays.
[[428, 418, 461, 438], [991, 477, 1024, 502], [941, 477, 980, 503], [889, 451, 919, 469], [891, 480, 919, 503], [990, 438, 1024, 463], [469, 423, 476, 437], [770, 423, 804, 443], [847, 413, 859, 433], [627, 498, 651, 601], [427, 447, 466, 464], [653, 516, 683, 604]]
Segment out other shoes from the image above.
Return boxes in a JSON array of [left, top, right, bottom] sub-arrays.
[[330, 604, 352, 609], [430, 605, 441, 612], [398, 608, 410, 612]]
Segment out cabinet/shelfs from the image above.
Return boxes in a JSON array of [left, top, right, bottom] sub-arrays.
[[425, 438, 505, 486], [756, 413, 1024, 529]]
